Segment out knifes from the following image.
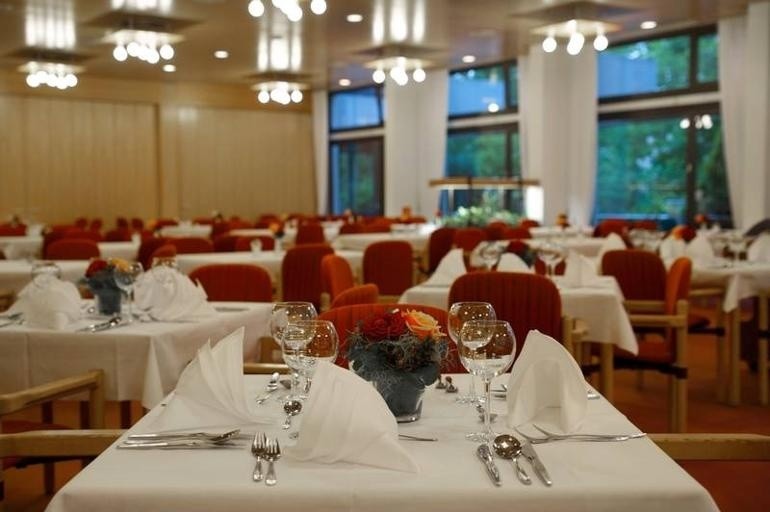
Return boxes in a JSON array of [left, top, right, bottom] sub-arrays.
[[77, 321, 108, 333], [490, 389, 503, 393], [477, 444, 500, 482], [129, 430, 256, 440], [520, 440, 553, 486], [117, 442, 247, 451]]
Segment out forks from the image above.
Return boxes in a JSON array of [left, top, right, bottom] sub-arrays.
[[251, 432, 267, 481], [446, 376, 457, 393], [532, 424, 647, 439], [500, 385, 592, 394], [515, 428, 629, 444], [256, 371, 279, 404], [436, 375, 445, 390], [264, 437, 282, 485]]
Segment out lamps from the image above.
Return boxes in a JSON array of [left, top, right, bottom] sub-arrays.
[[15, 60, 84, 91], [99, 24, 185, 65], [250, 78, 310, 106], [527, 16, 623, 56], [363, 54, 434, 86]]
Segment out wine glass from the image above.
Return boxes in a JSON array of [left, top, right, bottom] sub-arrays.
[[151, 258, 178, 325], [457, 321, 516, 444], [271, 301, 317, 400], [549, 248, 566, 283], [481, 249, 499, 272], [283, 319, 338, 380], [448, 301, 497, 405], [539, 242, 556, 277], [30, 260, 63, 331], [113, 261, 144, 325]]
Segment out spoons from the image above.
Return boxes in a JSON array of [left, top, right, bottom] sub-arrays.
[[281, 400, 300, 432], [91, 317, 122, 335], [493, 435, 532, 485], [124, 429, 242, 445]]
